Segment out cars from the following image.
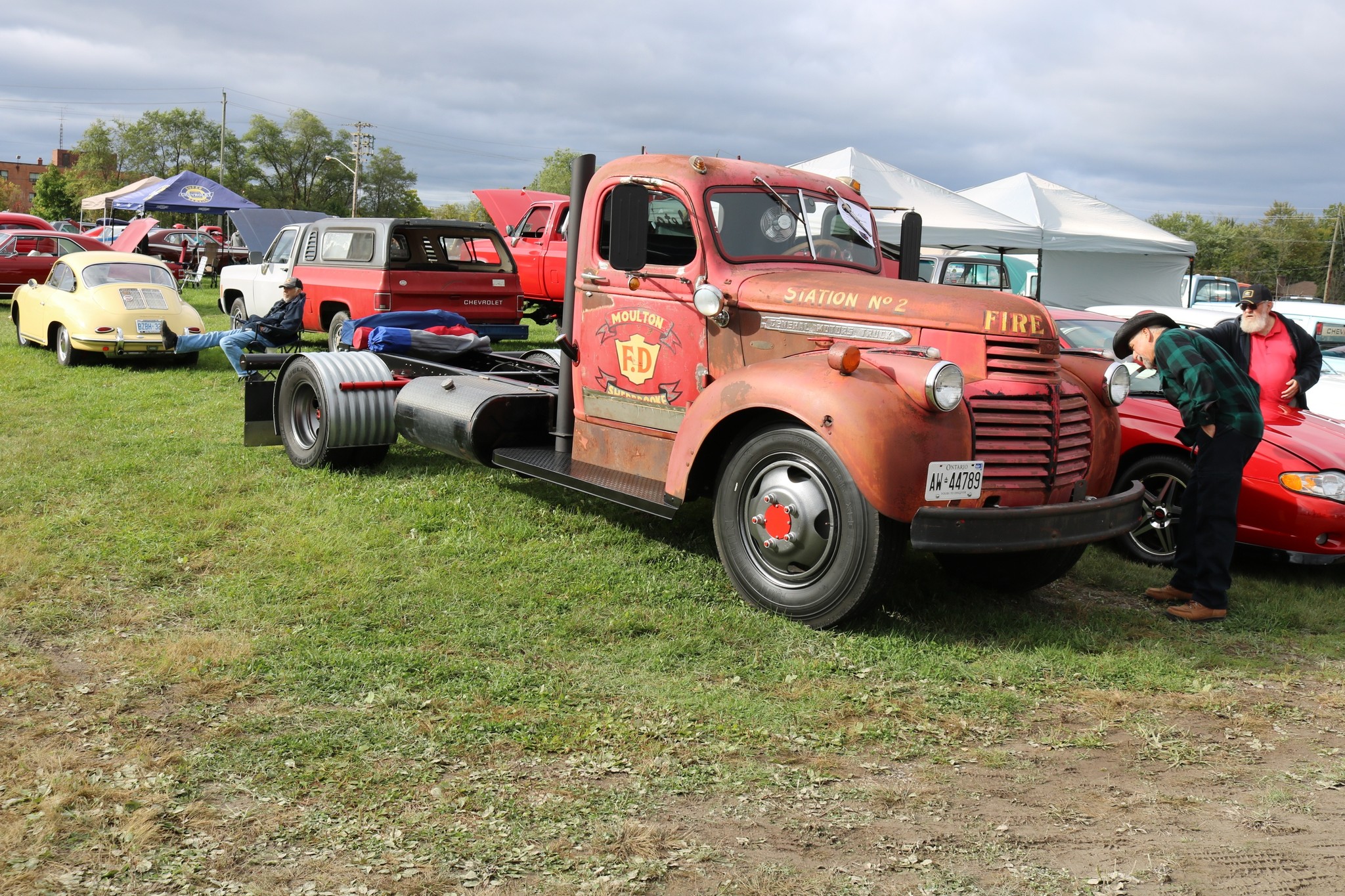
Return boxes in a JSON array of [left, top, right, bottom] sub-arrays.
[[9, 250, 206, 368], [1044, 304, 1345, 566], [918, 253, 1345, 423], [0, 212, 250, 277], [0, 229, 185, 300]]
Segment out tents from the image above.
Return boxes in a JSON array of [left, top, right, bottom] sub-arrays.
[[710, 147, 1197, 310], [111, 170, 262, 266], [80, 175, 200, 265]]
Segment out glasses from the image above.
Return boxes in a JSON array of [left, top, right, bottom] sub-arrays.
[[1240, 302, 1266, 310]]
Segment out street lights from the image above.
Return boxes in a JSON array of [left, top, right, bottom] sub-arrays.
[[325, 155, 359, 218]]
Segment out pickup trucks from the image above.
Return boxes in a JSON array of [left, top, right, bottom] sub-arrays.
[[459, 185, 900, 337], [216, 216, 530, 353]]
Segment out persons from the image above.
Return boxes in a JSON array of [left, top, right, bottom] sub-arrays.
[[133, 215, 149, 255], [161, 277, 306, 388], [1112, 310, 1266, 623], [231, 229, 246, 247], [1190, 284, 1323, 411]]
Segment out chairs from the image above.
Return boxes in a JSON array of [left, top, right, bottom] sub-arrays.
[[174, 239, 220, 292], [27, 250, 40, 256], [40, 252, 53, 257], [235, 315, 305, 381]]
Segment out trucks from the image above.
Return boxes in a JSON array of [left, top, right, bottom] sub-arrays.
[[239, 143, 1146, 631]]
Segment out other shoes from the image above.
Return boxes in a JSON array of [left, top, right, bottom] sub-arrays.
[[244, 372, 264, 381], [1165, 600, 1227, 623], [1144, 584, 1193, 603], [160, 320, 177, 350]]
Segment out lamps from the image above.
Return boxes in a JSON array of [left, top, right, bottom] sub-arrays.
[[17, 156, 20, 163]]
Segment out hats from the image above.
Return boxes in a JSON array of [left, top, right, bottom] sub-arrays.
[[279, 277, 303, 291], [1235, 283, 1272, 308], [1112, 309, 1181, 359]]
